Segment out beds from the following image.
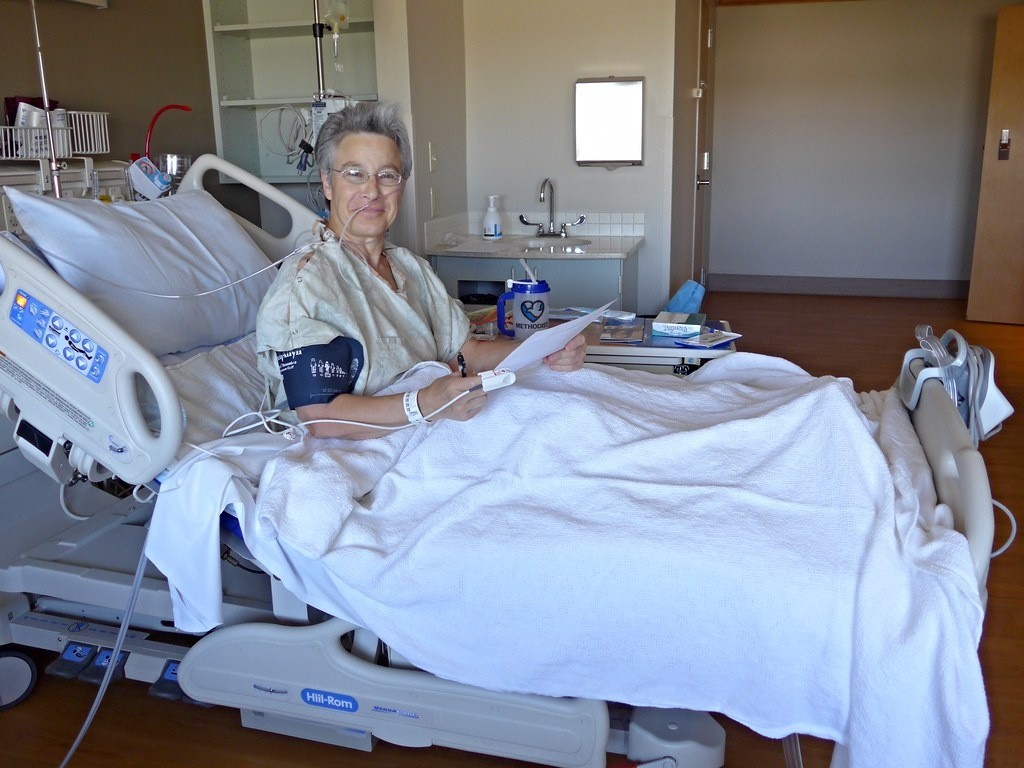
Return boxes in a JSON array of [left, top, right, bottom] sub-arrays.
[[1, 154, 995, 768]]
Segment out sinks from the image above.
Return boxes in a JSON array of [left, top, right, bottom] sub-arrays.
[[492, 237, 592, 247]]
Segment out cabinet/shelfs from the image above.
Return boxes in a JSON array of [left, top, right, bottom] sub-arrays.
[[436, 256, 638, 315], [202, 0, 376, 185]]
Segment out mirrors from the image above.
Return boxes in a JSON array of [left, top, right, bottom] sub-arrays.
[[574, 75, 645, 171]]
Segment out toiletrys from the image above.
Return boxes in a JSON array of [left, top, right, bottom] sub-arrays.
[[14, 100, 70, 158], [482, 194, 503, 240]]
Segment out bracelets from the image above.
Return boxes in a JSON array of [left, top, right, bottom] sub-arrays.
[[403, 390, 430, 427]]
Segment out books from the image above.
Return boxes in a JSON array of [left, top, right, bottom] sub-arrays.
[[599, 318, 646, 343]]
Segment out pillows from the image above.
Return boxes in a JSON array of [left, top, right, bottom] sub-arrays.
[[3, 184, 279, 360]]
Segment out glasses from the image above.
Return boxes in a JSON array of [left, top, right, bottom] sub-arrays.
[[325, 166, 406, 186]]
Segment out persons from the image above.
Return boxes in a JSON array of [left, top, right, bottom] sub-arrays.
[[256, 101, 587, 440]]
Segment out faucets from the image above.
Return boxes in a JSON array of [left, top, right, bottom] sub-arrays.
[[540, 177, 555, 233]]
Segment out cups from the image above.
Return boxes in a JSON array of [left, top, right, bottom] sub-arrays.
[[497, 280, 551, 341]]
